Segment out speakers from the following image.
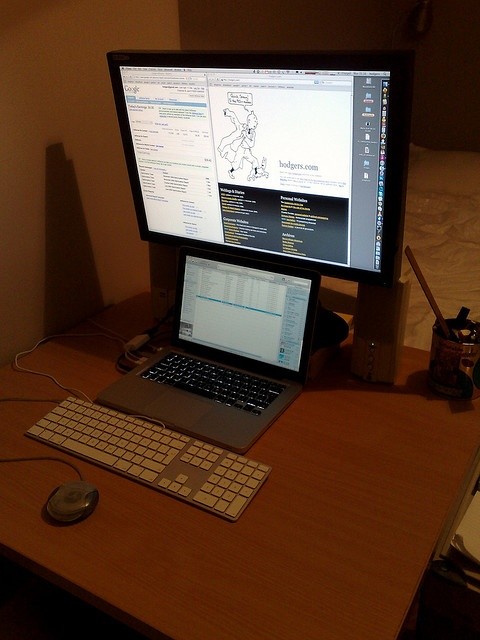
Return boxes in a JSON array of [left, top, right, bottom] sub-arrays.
[[348, 277, 414, 385], [147, 241, 181, 325]]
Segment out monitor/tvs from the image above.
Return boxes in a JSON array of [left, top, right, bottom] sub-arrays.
[[104, 49, 402, 288]]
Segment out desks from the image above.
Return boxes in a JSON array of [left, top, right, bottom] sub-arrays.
[[1, 292, 480, 640]]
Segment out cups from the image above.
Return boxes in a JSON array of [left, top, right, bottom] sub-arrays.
[[430, 319, 478, 400]]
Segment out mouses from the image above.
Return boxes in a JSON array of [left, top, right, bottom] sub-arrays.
[[40, 476, 100, 526]]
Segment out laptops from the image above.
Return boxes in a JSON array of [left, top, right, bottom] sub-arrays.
[[95, 245, 322, 456]]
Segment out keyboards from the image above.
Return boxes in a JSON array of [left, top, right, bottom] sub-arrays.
[[22, 394, 272, 522]]
